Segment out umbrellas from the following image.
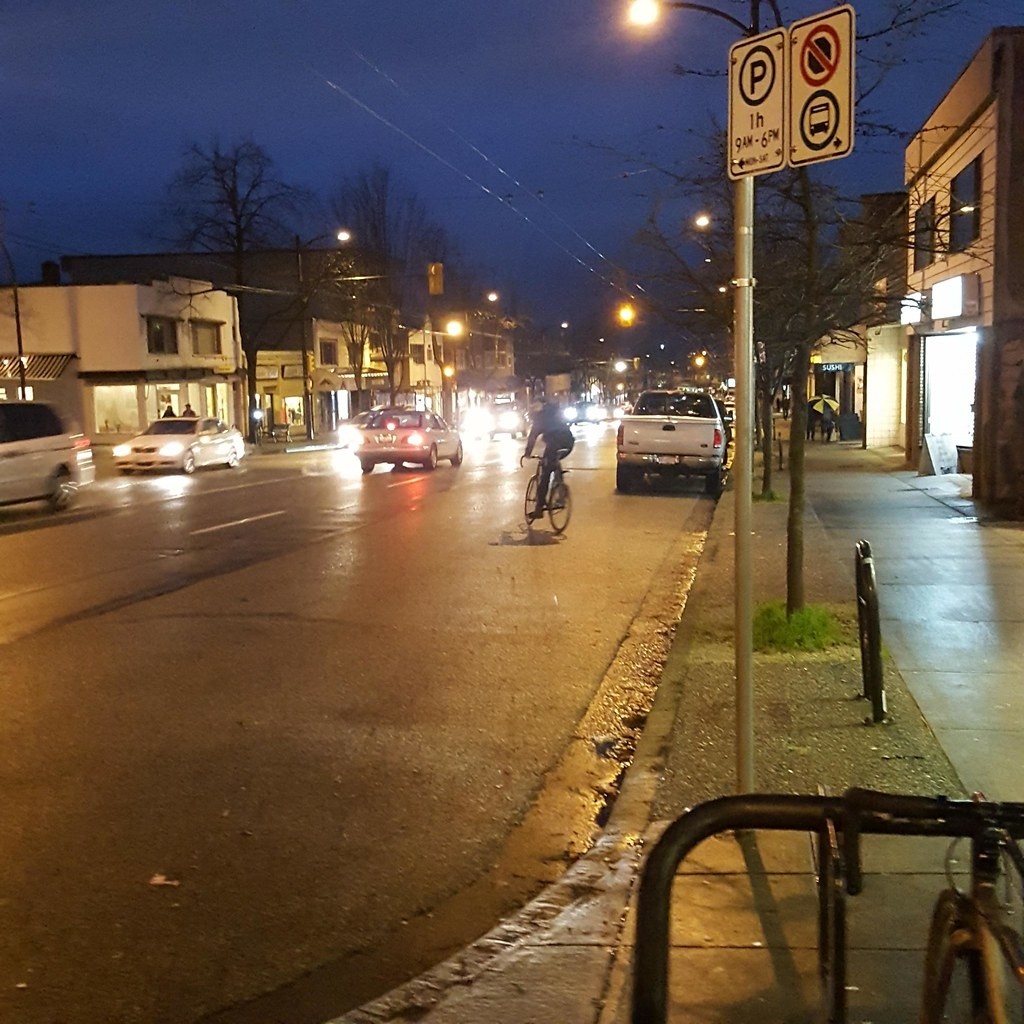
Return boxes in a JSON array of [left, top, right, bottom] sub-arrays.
[[807, 393, 840, 414]]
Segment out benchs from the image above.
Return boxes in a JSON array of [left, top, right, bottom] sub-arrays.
[[261, 423, 293, 442]]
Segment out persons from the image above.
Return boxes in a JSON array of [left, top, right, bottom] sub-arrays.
[[782, 395, 789, 419], [525, 397, 573, 518], [163, 405, 176, 417], [183, 404, 195, 417], [806, 403, 835, 442]]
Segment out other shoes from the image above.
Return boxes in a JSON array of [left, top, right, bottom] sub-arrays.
[[529, 512, 544, 519]]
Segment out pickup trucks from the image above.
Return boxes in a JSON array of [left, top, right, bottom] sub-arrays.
[[617, 390, 726, 495]]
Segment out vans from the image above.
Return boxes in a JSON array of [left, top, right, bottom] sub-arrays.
[[0, 400, 94, 515]]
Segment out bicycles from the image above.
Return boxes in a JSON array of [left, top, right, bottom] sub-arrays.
[[521, 447, 571, 533], [841, 786, 1024, 1024]]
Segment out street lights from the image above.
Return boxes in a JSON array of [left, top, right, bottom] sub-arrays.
[[297, 229, 350, 440], [635, 0, 759, 795]]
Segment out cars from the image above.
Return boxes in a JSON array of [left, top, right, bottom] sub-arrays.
[[608, 404, 634, 419], [566, 402, 604, 423], [711, 397, 735, 443], [352, 407, 463, 472], [115, 417, 244, 474], [487, 402, 532, 438]]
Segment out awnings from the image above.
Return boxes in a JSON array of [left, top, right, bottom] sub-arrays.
[[0, 353, 80, 381]]
